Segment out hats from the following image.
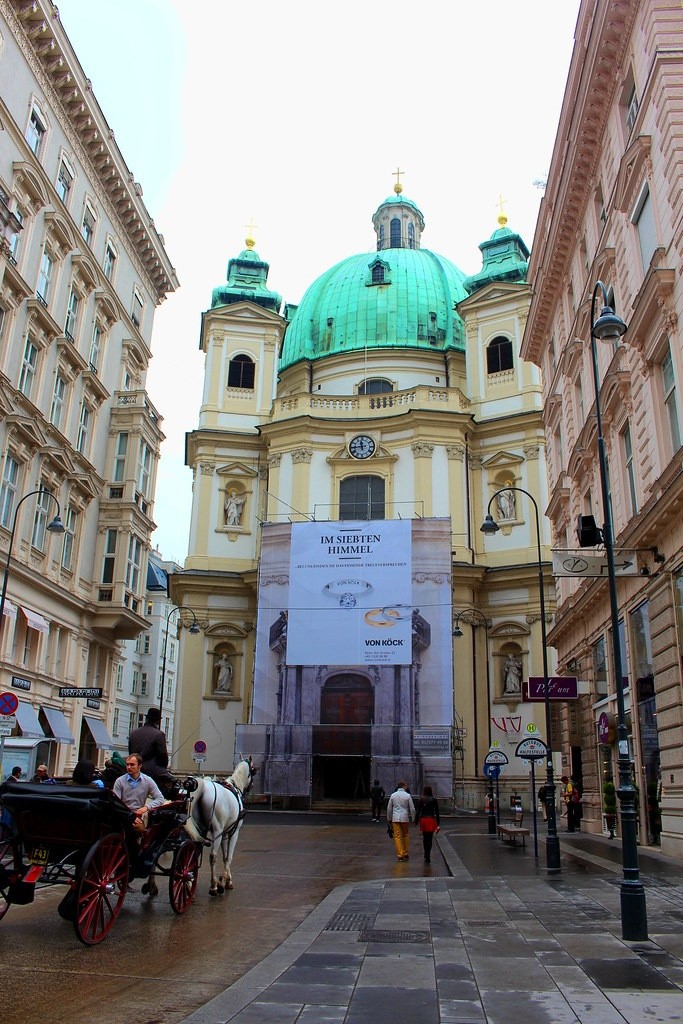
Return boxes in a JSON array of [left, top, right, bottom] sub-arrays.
[[144, 708, 162, 720], [37, 765, 47, 771]]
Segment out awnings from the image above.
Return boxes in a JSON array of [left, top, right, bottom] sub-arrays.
[[15, 702, 45, 738], [84, 717, 113, 750], [41, 706, 74, 744]]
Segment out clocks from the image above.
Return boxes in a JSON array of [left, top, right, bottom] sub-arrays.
[[348, 434, 376, 459]]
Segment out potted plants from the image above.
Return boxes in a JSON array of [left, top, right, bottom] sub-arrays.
[[603, 782, 618, 832]]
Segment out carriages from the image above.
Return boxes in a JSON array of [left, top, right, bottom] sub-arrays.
[[1, 751, 255, 946]]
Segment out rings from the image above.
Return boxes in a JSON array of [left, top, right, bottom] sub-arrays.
[[323, 578, 374, 608], [382, 602, 411, 622], [364, 608, 400, 627]]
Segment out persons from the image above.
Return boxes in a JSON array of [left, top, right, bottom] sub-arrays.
[[370, 780, 386, 822], [504, 654, 521, 693], [72, 760, 104, 788], [31, 765, 50, 781], [491, 483, 515, 519], [386, 781, 440, 863], [128, 708, 177, 790], [560, 776, 577, 833], [113, 753, 164, 834], [538, 781, 548, 822], [226, 491, 246, 526], [6, 767, 21, 782], [216, 653, 233, 691]]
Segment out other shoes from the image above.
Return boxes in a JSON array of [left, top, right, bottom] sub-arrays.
[[376, 819, 379, 823], [425, 859, 430, 864], [405, 856, 409, 860], [372, 819, 376, 821], [399, 857, 403, 861]]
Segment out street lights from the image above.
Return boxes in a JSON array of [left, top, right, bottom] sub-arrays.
[[0, 490, 65, 624], [480, 487, 562, 875], [452, 608, 497, 834], [160, 607, 201, 732], [585, 279, 650, 942]]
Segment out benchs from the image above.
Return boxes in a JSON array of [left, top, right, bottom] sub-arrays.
[[496, 823, 529, 849]]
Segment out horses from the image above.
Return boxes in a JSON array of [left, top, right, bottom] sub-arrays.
[[148, 752, 257, 898]]
